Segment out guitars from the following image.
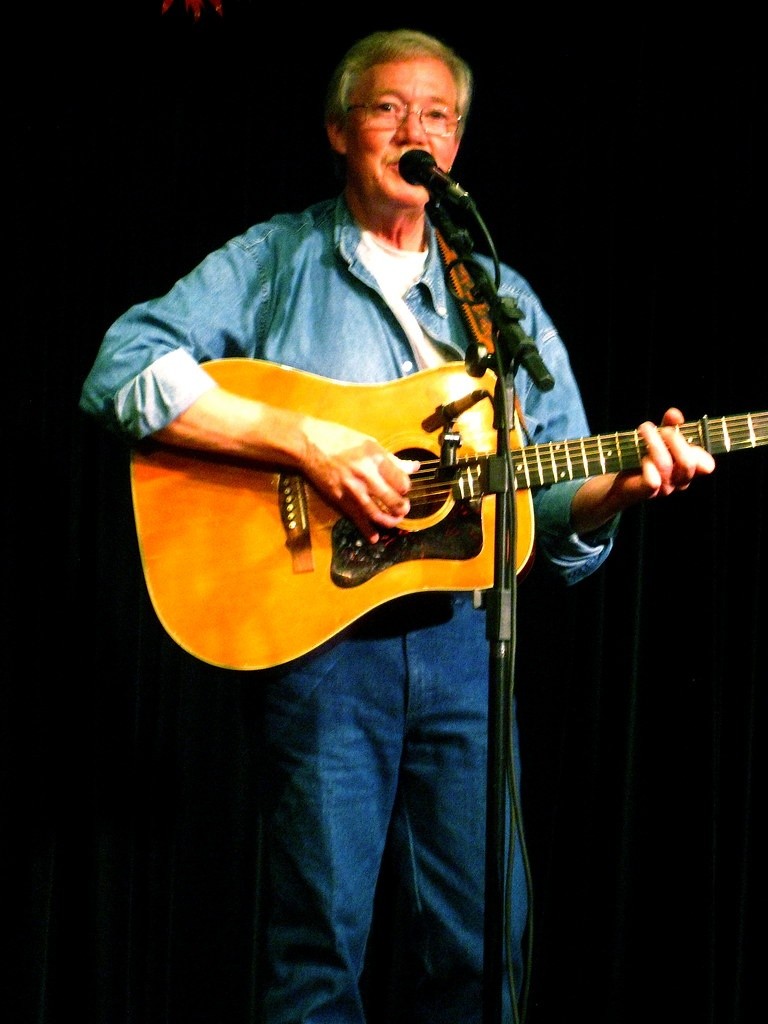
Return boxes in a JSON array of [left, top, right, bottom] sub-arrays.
[[132, 360, 768, 672]]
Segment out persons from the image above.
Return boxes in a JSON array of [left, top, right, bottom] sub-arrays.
[[77, 29, 718, 1023]]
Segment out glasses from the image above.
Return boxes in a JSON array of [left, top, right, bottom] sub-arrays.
[[345, 90, 462, 138]]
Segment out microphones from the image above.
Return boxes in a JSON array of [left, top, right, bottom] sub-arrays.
[[398, 150, 474, 209]]
